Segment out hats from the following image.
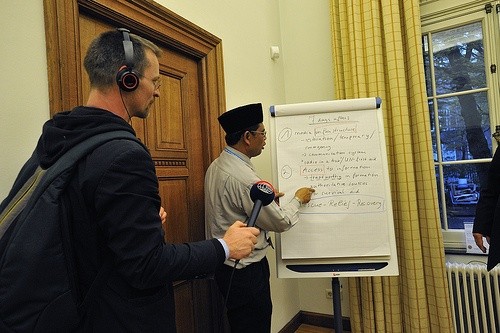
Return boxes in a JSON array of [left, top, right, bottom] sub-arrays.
[[217, 103, 263, 134]]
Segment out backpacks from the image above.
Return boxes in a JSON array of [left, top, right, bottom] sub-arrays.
[[0, 131, 156, 333]]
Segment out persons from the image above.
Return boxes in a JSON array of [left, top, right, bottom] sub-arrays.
[[472, 143, 500, 271], [11, 29, 260, 333], [203, 102, 316, 333]]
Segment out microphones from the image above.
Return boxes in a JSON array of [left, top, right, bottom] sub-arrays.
[[235, 181, 275, 264]]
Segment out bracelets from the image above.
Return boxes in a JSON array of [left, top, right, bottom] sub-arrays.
[[294, 196, 302, 205]]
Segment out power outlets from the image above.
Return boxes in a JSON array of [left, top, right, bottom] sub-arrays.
[[326, 289, 333, 299]]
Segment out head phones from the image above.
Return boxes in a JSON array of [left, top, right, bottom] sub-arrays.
[[115, 27, 139, 92]]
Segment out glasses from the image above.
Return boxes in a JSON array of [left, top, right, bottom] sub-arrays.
[[248, 130, 267, 136], [131, 70, 161, 85]]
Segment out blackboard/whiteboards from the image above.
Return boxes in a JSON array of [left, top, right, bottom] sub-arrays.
[[270, 98, 399, 279]]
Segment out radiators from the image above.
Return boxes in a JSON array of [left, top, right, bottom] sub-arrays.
[[445, 262, 500, 333]]
